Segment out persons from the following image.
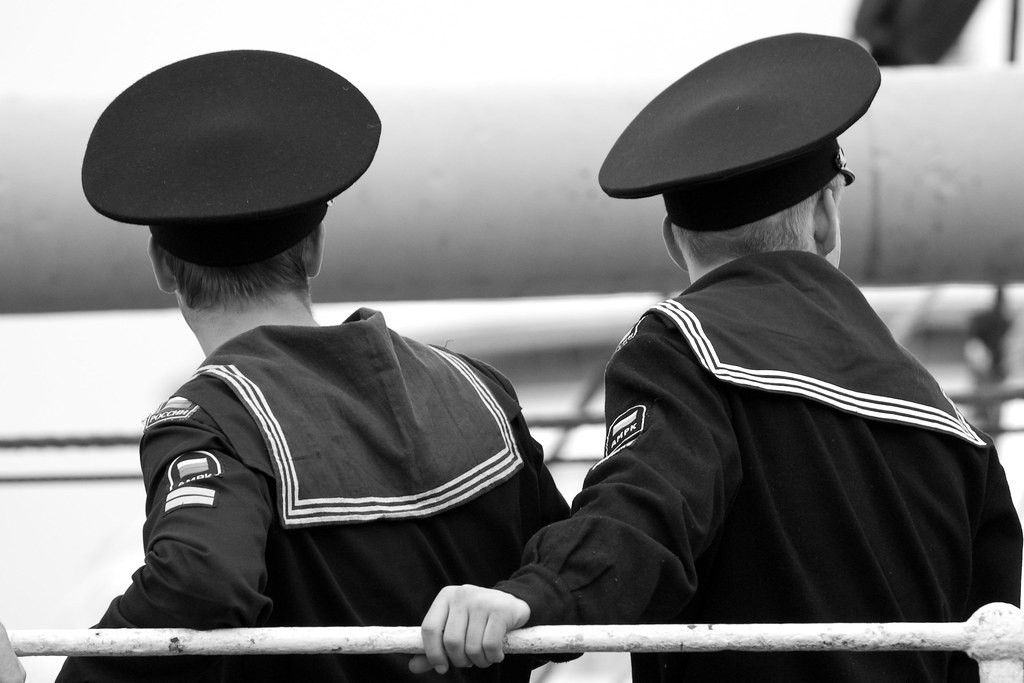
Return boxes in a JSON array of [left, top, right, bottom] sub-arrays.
[[407, 31, 1023, 683], [965, 278, 1016, 449], [52, 48, 585, 683]]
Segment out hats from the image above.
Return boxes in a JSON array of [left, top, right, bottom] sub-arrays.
[[81, 50, 381, 269], [595, 32, 881, 234]]
